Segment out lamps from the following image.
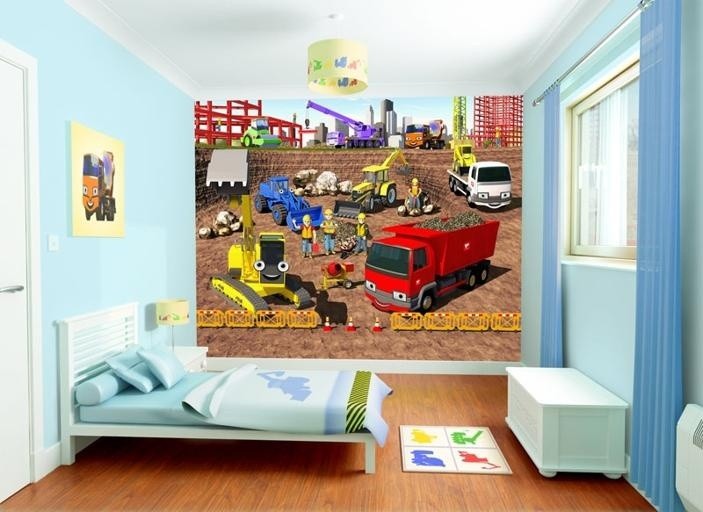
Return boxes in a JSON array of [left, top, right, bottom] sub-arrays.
[[303, 10, 373, 94], [154, 298, 189, 347]]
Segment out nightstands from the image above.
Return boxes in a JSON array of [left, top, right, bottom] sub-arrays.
[[175, 346, 210, 372]]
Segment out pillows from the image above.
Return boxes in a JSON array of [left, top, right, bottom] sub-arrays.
[[74, 369, 131, 406], [137, 343, 188, 391], [104, 347, 161, 394]]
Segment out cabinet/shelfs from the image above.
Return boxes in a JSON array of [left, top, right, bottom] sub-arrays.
[[501, 364, 630, 481]]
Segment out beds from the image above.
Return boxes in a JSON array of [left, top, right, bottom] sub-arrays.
[[52, 301, 393, 477]]
